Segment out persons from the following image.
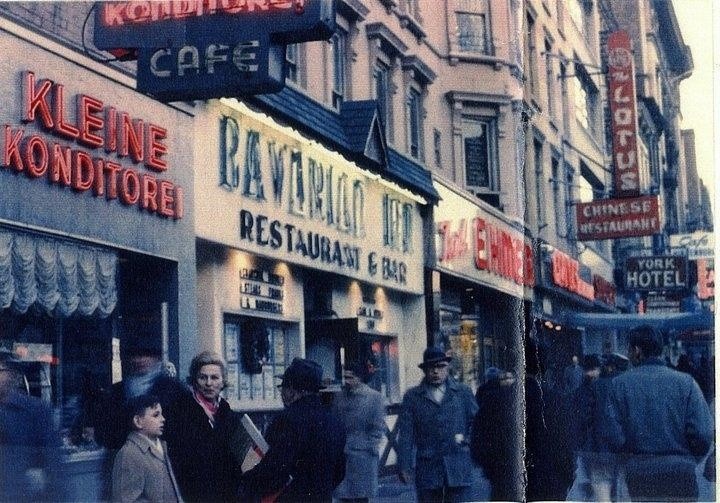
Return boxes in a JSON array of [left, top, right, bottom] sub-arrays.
[[396, 325, 717, 502], [96, 348, 387, 502], [0, 352, 78, 503]]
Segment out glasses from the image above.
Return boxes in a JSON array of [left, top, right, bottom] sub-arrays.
[[277, 384, 284, 391]]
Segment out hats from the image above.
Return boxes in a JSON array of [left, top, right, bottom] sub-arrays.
[[608, 351, 629, 371], [417, 346, 453, 368], [273, 357, 326, 390]]
